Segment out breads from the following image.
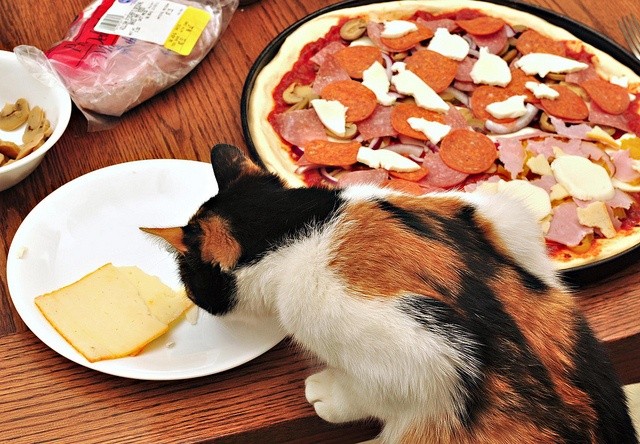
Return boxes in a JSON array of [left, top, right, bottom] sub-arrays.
[[32, 262, 197, 362]]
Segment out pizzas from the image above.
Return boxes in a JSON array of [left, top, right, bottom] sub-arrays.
[[249, 0, 639, 273]]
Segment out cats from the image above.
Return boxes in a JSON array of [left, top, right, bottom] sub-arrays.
[[139, 142, 640, 438]]
